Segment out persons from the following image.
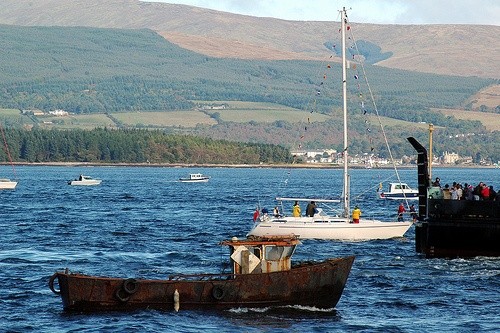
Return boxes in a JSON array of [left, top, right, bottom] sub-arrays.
[[427, 174, 500, 200], [397, 203, 406, 222], [306, 201, 317, 217], [409, 204, 418, 221], [292, 202, 301, 217], [351, 205, 362, 223], [273, 207, 281, 218]]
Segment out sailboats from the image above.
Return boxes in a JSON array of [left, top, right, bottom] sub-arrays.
[[0, 125, 18, 189], [246, 6, 412, 239]]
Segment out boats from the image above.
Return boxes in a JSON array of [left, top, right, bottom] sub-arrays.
[[179, 173, 211, 183], [376, 183, 419, 200], [58, 233, 354, 315], [408, 124, 500, 259], [68, 174, 103, 186]]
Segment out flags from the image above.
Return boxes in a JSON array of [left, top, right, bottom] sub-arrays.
[[253, 208, 260, 222], [283, 15, 385, 199]]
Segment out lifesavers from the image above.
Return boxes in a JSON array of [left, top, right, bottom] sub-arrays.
[[124, 278, 137, 294], [213, 285, 225, 300], [117, 287, 130, 301], [50, 273, 60, 294]]
